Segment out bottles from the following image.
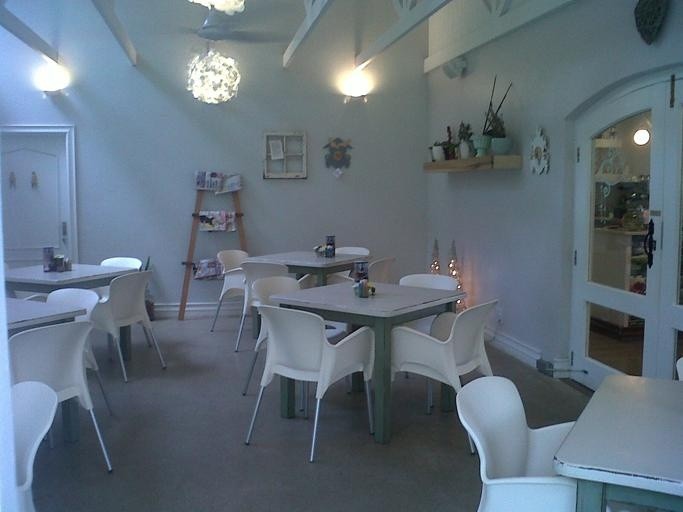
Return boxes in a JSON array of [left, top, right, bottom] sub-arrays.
[[326, 235, 335, 256], [358, 278, 376, 298], [42, 247, 72, 272]]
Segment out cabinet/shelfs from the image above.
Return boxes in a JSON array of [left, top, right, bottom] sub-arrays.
[[589, 226, 648, 342], [592, 137, 626, 185]]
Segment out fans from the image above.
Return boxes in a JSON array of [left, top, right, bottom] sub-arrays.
[[157, 1, 291, 48]]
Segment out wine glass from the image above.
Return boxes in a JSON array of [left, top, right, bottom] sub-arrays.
[[473, 136, 490, 158]]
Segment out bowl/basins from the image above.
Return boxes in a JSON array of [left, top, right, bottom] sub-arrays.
[[492, 138, 512, 155]]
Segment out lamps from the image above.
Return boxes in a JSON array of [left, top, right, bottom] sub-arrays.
[[334, 68, 375, 106], [185, 41, 240, 103], [32, 64, 70, 100], [190, 2, 245, 15]]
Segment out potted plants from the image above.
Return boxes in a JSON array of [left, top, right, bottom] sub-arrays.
[[425, 105, 511, 164]]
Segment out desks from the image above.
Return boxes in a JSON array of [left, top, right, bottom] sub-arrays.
[[552, 367, 682, 511]]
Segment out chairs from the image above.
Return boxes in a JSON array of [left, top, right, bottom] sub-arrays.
[[675, 357, 681, 380], [2, 254, 167, 511], [240, 274, 468, 462], [385, 299, 499, 456], [452, 374, 612, 511], [210, 246, 395, 397]]
